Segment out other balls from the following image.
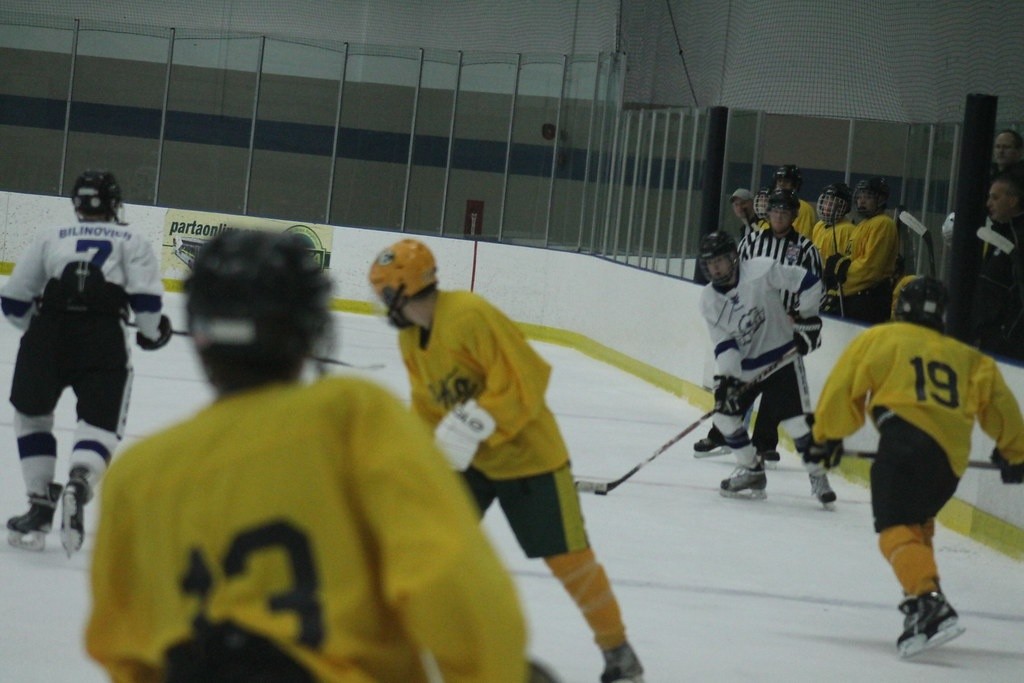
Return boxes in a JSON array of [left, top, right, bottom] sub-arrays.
[[594, 490, 607, 495]]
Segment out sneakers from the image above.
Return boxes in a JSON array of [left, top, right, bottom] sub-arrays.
[[897, 578, 967, 654], [60, 476, 87, 552], [692, 424, 732, 458], [809, 471, 836, 511], [601, 639, 645, 683], [719, 463, 767, 499], [6, 481, 62, 551]]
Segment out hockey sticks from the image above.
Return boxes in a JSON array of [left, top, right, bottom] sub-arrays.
[[574, 344, 801, 493], [126, 322, 386, 370], [976, 226, 1018, 263], [898, 210, 936, 279], [841, 448, 1000, 469], [831, 201, 845, 318]]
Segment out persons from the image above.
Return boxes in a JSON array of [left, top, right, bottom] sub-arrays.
[[0, 172, 174, 560], [82, 230, 530, 683], [809, 276, 1024, 661], [368, 240, 646, 683], [695, 130, 1024, 508]]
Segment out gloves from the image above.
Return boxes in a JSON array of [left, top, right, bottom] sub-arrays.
[[991, 447, 1024, 484], [135, 315, 172, 351], [712, 376, 747, 415], [820, 290, 838, 314], [823, 252, 851, 284], [793, 315, 823, 356], [803, 437, 844, 478]]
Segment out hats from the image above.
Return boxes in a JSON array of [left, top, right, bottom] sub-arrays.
[[729, 188, 752, 205]]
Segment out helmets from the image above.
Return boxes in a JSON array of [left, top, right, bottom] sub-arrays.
[[371, 237, 439, 329], [895, 276, 949, 333], [698, 230, 740, 285], [853, 176, 891, 217], [187, 232, 333, 354], [816, 179, 852, 223], [753, 163, 803, 220], [72, 170, 123, 222]]
